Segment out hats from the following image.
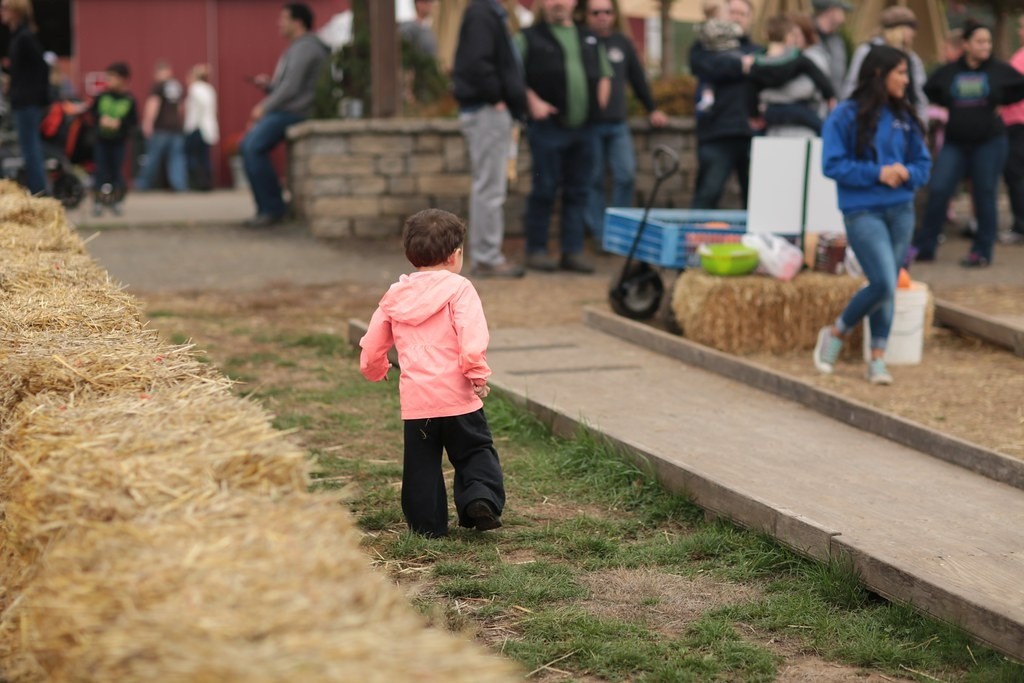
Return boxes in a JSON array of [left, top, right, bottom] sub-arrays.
[[880, 5, 917, 29], [813, 0, 852, 12]]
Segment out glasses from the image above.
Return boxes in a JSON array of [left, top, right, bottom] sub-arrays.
[[583, 8, 613, 17]]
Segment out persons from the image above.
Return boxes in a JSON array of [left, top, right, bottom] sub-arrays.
[[0, 0, 50, 198], [394, 0, 438, 57], [446, 0, 668, 278], [239, 2, 335, 229], [842, 4, 930, 133], [358, 208, 506, 539], [130, 58, 191, 192], [87, 63, 140, 199], [996, 14, 1024, 245], [813, 46, 934, 386], [912, 19, 1024, 266], [181, 62, 221, 191], [685, 0, 855, 208]]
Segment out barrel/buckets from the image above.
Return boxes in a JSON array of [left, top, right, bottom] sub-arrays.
[[862, 280, 930, 365]]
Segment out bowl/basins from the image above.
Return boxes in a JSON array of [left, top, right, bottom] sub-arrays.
[[695, 243, 761, 277]]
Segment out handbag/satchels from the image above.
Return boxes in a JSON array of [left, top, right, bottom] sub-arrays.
[[742, 230, 805, 282]]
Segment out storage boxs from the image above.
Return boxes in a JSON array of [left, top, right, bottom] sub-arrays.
[[602, 207, 746, 267]]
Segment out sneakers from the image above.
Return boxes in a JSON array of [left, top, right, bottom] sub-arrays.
[[814, 324, 843, 373], [865, 359, 892, 387]]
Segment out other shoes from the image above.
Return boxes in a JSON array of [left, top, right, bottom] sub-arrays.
[[467, 500, 502, 532], [907, 246, 934, 261], [958, 251, 988, 268], [592, 229, 609, 256], [525, 251, 561, 271], [243, 207, 287, 228], [559, 256, 595, 275], [469, 258, 524, 278]]
